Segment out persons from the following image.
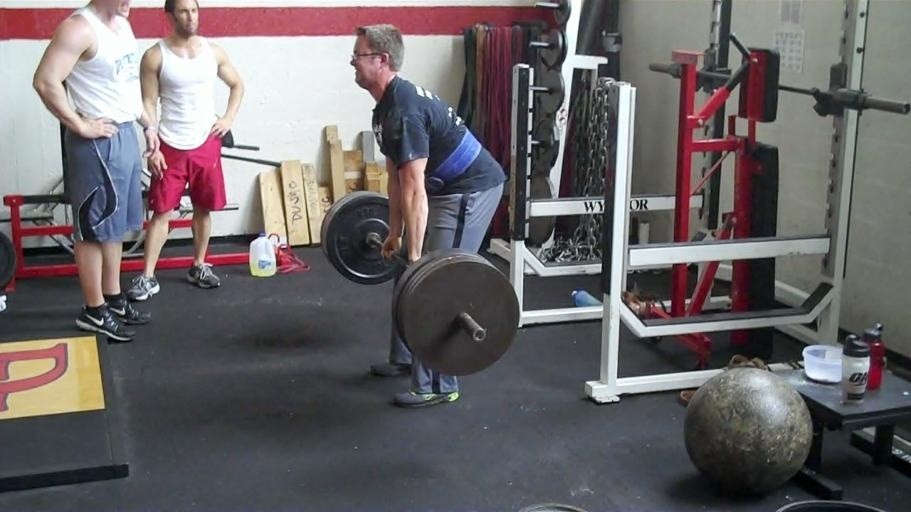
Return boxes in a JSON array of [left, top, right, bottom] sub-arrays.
[[124, 0, 247, 303], [30, 0, 161, 343], [348, 23, 508, 408]]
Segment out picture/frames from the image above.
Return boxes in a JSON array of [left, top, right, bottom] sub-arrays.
[[768, 366, 909, 498]]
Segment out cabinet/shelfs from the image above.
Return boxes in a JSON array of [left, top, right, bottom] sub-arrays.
[[567, 288, 608, 310], [840, 333, 870, 403], [859, 324, 888, 390]]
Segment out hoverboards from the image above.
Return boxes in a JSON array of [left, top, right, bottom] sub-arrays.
[[319, 192, 520, 376]]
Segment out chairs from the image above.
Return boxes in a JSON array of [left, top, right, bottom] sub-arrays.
[[369, 360, 412, 378], [394, 388, 460, 409], [106, 297, 153, 326], [126, 272, 160, 302], [186, 261, 220, 289], [76, 304, 137, 341]]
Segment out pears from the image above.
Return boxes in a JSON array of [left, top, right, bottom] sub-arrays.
[[249, 232, 277, 276]]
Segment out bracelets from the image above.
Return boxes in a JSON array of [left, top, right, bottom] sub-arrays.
[[800, 346, 842, 385]]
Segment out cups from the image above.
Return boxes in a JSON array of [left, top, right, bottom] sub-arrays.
[[349, 51, 385, 62]]
